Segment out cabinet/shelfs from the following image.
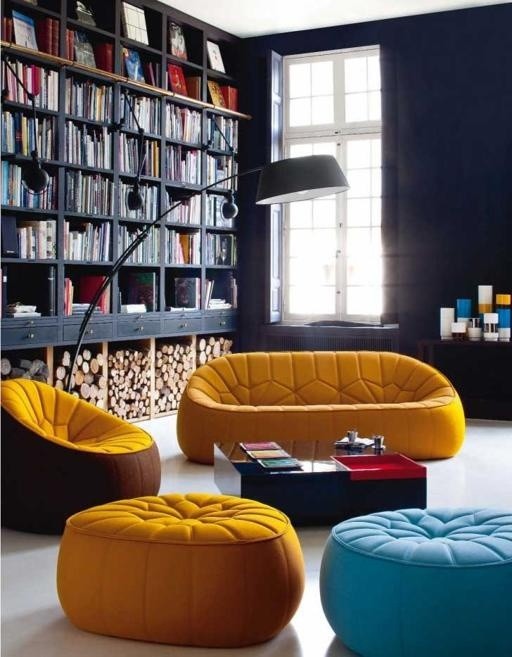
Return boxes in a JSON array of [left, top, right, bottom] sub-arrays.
[[0, 0, 246, 349]]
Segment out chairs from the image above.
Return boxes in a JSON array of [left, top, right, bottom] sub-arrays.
[[1, 378, 161, 536]]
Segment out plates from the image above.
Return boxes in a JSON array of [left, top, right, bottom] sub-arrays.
[[372, 445, 385, 449]]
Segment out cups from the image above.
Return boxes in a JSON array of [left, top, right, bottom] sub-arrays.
[[440, 285, 511, 342], [373, 435, 381, 447], [347, 430, 358, 442]]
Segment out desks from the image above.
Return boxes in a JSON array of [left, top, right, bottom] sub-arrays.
[[417, 339, 512, 365]]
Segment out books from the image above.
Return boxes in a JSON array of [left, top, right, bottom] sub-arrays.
[[118, 1, 162, 265], [205, 41, 238, 310], [64, 2, 114, 317], [165, 20, 202, 265], [2, 1, 57, 319]]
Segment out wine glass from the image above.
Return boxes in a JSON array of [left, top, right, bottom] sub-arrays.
[[221, 241, 227, 264]]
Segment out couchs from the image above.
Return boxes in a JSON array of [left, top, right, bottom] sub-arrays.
[[176, 350, 465, 463]]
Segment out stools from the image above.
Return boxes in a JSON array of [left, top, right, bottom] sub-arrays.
[[57, 493, 304, 648], [320, 506, 512, 657]]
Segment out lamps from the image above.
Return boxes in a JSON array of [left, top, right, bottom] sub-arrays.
[[123, 88, 147, 210], [211, 114, 240, 221], [65, 155, 350, 393], [5, 56, 50, 195]]
